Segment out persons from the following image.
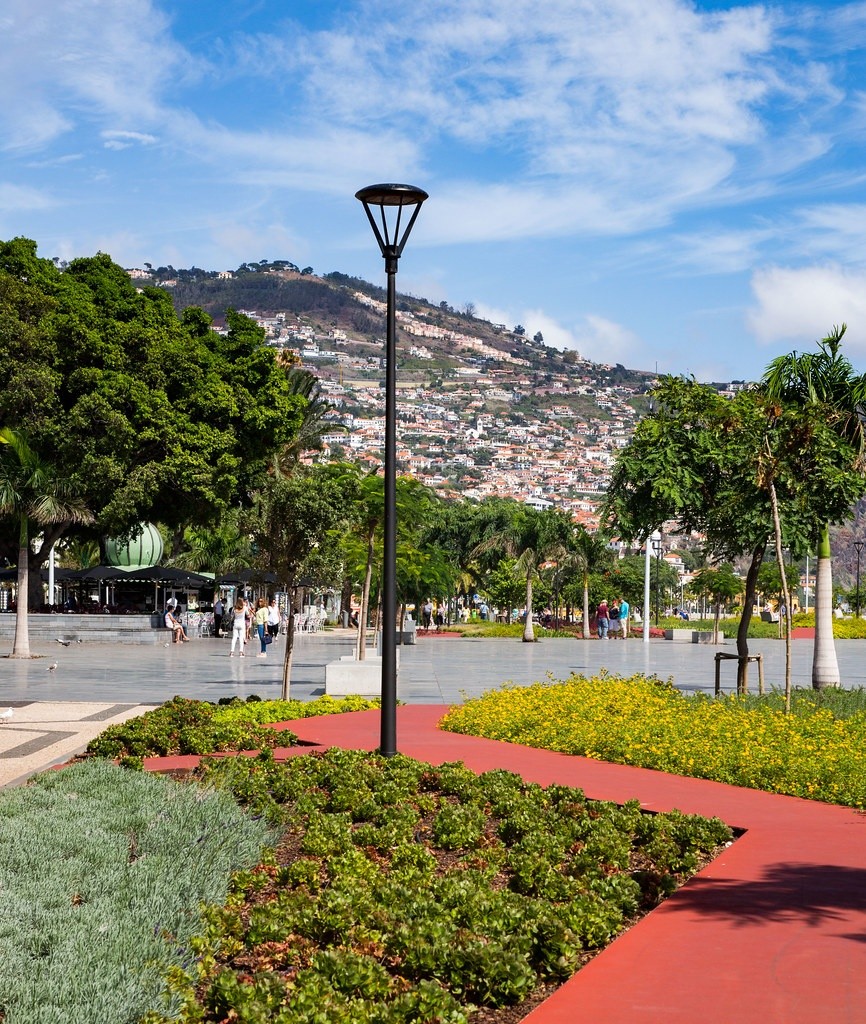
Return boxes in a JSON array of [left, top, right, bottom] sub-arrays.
[[220, 597, 225, 614], [320, 601, 327, 622], [422, 598, 433, 630], [662, 601, 850, 619], [173, 605, 187, 636], [594, 600, 610, 640], [436, 604, 444, 626], [165, 606, 188, 644], [256, 598, 268, 657], [348, 608, 359, 630], [213, 598, 227, 637], [243, 596, 255, 638], [6, 591, 180, 620], [457, 599, 567, 625], [618, 598, 629, 639], [608, 599, 620, 639], [229, 597, 249, 658], [267, 598, 280, 641]]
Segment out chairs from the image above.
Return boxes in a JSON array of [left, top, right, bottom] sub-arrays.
[[277, 613, 323, 636], [181, 611, 215, 638]]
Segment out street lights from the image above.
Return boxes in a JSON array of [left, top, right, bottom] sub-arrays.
[[853, 541, 864, 618], [354, 181, 430, 757]]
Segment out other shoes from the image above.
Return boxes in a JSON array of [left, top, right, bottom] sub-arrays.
[[609, 636, 612, 639], [176, 640, 183, 643], [229, 652, 235, 657], [598, 636, 602, 639], [603, 636, 608, 640], [215, 635, 223, 638], [240, 652, 245, 657], [620, 637, 627, 640], [183, 637, 190, 641], [614, 637, 617, 639], [256, 653, 266, 657]]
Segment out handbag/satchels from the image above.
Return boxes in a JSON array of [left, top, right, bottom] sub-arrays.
[[173, 621, 181, 630], [261, 632, 273, 644]]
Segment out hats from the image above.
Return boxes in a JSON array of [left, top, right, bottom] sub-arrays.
[[601, 600, 608, 603], [766, 603, 773, 608]]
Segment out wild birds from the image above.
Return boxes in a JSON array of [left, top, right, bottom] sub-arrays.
[[46, 661, 59, 673], [163, 641, 170, 648], [55, 637, 83, 648]]
